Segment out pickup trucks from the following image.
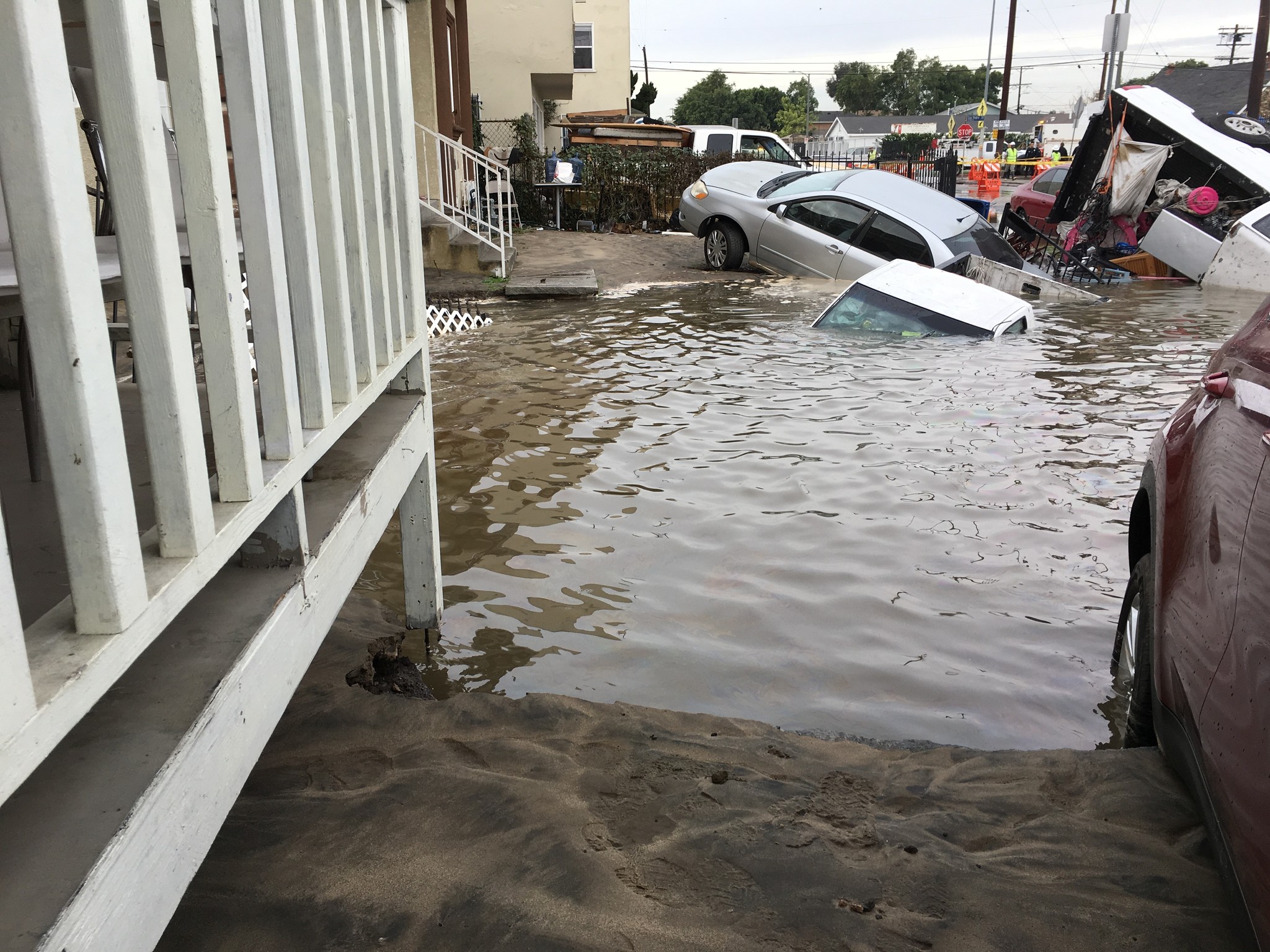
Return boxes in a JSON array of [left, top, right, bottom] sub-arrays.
[[935, 140, 1032, 176], [808, 251, 1113, 341], [675, 125, 839, 172]]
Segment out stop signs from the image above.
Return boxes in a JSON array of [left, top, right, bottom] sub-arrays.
[[957, 124, 973, 140]]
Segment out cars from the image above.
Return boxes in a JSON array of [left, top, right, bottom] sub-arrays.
[[679, 161, 1056, 280], [1012, 84, 1270, 293], [806, 145, 880, 170], [1107, 290, 1270, 952]]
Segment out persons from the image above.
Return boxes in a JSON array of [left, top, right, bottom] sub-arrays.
[[1051, 145, 1061, 166], [867, 146, 879, 164], [1059, 142, 1067, 161], [1025, 142, 1038, 178], [1072, 141, 1081, 156], [1036, 142, 1042, 160], [1003, 141, 1018, 181]]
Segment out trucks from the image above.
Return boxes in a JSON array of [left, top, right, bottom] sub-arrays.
[[1031, 123, 1077, 161]]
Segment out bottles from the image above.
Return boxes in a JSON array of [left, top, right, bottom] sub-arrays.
[[545, 151, 562, 183], [567, 152, 584, 183]]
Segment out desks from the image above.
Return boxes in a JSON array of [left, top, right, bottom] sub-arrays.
[[0, 231, 249, 483], [534, 183, 583, 230]]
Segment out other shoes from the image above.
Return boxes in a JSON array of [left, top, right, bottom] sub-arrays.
[[1004, 179, 1007, 180], [1025, 176, 1027, 177], [1012, 177, 1016, 180]]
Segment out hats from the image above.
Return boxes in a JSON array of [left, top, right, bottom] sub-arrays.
[[1052, 145, 1059, 150]]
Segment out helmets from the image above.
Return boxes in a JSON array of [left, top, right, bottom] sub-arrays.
[[1010, 142, 1015, 145]]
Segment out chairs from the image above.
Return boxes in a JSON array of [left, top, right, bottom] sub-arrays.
[[486, 181, 522, 230], [82, 115, 258, 384]]
